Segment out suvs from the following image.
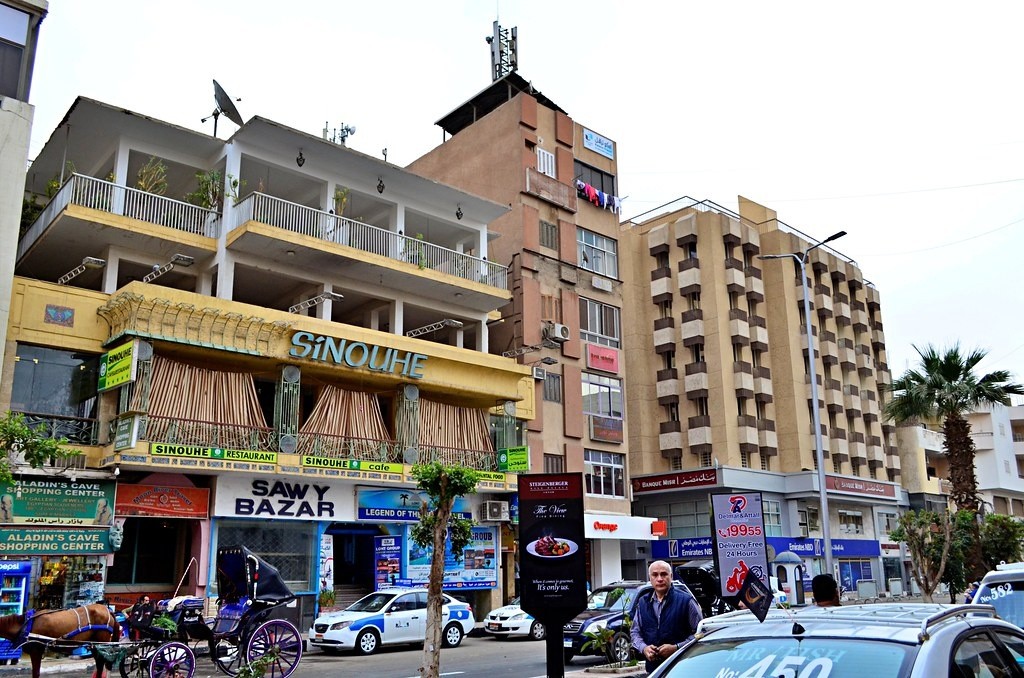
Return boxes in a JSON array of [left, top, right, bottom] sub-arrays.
[[562, 579, 704, 664]]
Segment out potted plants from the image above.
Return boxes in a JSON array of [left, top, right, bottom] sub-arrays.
[[318, 591, 338, 614]]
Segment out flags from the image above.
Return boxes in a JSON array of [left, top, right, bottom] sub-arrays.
[[736, 567, 774, 623]]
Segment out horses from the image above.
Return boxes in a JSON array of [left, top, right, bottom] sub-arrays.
[[0, 604, 120, 678]]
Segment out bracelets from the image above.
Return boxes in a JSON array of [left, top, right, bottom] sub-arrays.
[[676, 644, 680, 650]]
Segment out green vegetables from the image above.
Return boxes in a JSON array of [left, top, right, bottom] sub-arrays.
[[549, 542, 566, 550], [152, 612, 177, 633]]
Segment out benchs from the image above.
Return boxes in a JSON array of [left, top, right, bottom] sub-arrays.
[[212, 594, 275, 634], [142, 596, 203, 632]]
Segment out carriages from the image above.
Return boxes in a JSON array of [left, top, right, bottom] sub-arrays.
[[0, 543, 303, 678]]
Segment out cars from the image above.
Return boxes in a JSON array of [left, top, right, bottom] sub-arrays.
[[965, 560, 1024, 633], [308, 586, 476, 657], [482, 586, 605, 641], [646, 603, 1024, 678]]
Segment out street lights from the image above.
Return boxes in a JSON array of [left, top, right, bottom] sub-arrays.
[[757, 230, 847, 579]]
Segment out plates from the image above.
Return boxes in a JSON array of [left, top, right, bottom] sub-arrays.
[[526, 538, 579, 560]]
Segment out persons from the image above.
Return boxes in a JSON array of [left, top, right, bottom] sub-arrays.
[[812, 574, 842, 607], [961, 643, 1012, 678], [515, 561, 520, 596], [738, 600, 749, 610], [97, 595, 154, 641], [964, 582, 980, 617], [630, 561, 704, 678]]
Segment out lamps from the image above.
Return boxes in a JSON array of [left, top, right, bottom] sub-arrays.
[[56, 256, 106, 285], [296, 148, 305, 167], [456, 293, 462, 296], [289, 293, 345, 315], [288, 251, 296, 256], [377, 176, 386, 194], [581, 250, 589, 268], [142, 253, 195, 283], [524, 357, 558, 367], [457, 203, 463, 220], [406, 320, 463, 339], [503, 342, 560, 358]]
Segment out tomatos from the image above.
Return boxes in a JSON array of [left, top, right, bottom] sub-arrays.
[[554, 544, 569, 554]]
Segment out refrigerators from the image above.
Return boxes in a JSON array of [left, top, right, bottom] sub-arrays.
[[0, 559, 33, 664]]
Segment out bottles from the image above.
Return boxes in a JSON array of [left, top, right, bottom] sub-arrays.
[[1, 592, 21, 603], [3, 576, 23, 588], [0, 606, 20, 617]]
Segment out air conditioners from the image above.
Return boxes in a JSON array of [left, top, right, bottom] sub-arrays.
[[533, 366, 546, 380], [483, 500, 511, 520], [550, 323, 569, 342]]
